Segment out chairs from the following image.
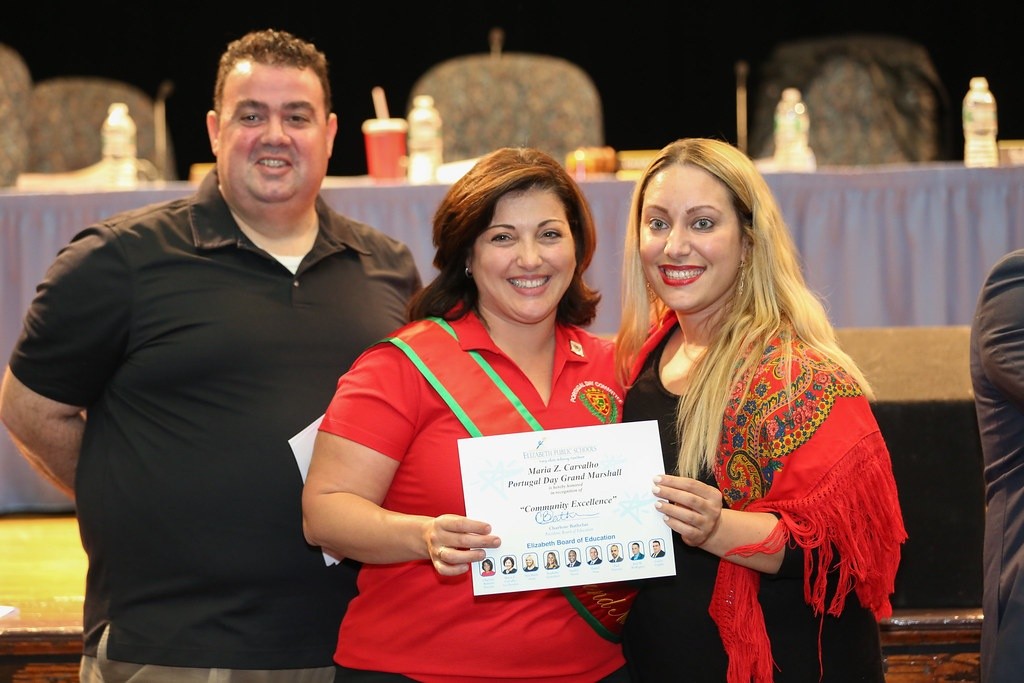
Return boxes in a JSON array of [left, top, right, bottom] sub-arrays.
[[407, 54, 601, 167], [751, 37, 938, 164], [22, 81, 171, 179]]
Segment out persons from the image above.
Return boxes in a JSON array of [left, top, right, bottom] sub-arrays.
[[301, 150, 634, 683], [970, 250, 1024, 683], [0, 29, 424, 683], [482, 542, 665, 578], [619, 137, 898, 683]]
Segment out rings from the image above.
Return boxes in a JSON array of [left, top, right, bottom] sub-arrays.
[[436, 548, 445, 556]]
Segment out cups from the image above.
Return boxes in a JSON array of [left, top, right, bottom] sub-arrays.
[[362, 118, 408, 179], [999, 140, 1024, 166]]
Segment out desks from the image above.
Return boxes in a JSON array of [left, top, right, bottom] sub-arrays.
[[834, 326, 976, 408], [0, 518, 88, 683], [0, 161, 1024, 512]]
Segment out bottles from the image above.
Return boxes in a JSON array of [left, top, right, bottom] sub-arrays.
[[408, 95, 443, 186], [963, 77, 998, 167], [775, 89, 816, 173], [100, 103, 136, 187]]
